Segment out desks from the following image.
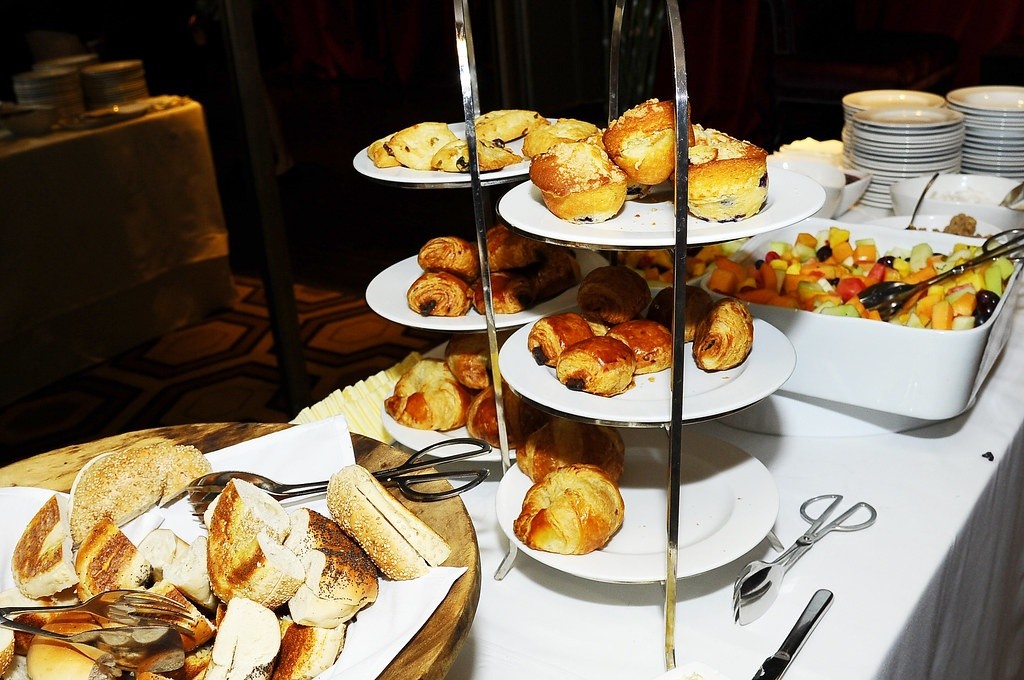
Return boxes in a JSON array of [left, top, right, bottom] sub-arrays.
[[391, 204, 1024, 680], [0, 94, 241, 410]]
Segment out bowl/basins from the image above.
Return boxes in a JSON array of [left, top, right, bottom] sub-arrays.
[[0, 101, 63, 134], [576, 153, 1024, 420]]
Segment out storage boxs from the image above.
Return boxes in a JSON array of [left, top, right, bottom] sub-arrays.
[[698, 217, 1024, 422]]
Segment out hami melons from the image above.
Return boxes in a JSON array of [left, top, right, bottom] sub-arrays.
[[711, 233, 975, 329]]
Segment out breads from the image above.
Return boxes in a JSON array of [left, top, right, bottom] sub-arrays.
[[367, 109, 599, 171], [407, 224, 580, 317], [529, 99, 768, 224], [384, 334, 624, 554], [0, 436, 450, 680], [529, 267, 754, 396]]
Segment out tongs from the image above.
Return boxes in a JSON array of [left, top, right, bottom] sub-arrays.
[[186, 438, 493, 529], [857, 228, 1024, 322], [0, 590, 195, 672], [732, 495, 877, 625]]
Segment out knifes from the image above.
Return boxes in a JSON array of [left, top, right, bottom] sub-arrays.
[[752, 589, 835, 680]]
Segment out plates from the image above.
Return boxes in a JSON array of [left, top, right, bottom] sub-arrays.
[[0, 422, 482, 680], [498, 164, 826, 246], [381, 390, 517, 460], [495, 429, 780, 585], [498, 287, 797, 423], [353, 118, 601, 183], [365, 250, 610, 330], [13, 53, 149, 109], [840, 85, 1024, 208]]
